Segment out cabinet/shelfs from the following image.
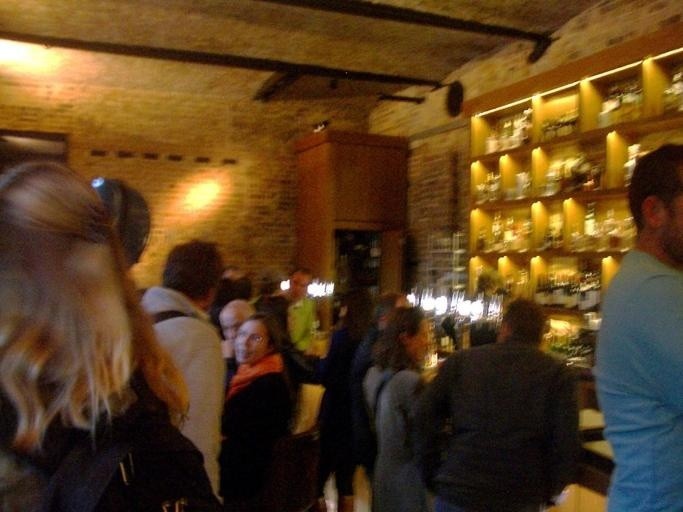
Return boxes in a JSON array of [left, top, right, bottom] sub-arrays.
[[463, 21, 683, 361], [290, 127, 408, 293]]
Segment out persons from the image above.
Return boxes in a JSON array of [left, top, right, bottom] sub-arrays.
[[1, 160, 225, 512], [133, 236, 226, 510], [207, 259, 432, 512], [80, 173, 152, 277], [430, 297, 582, 512], [593, 142, 683, 512]]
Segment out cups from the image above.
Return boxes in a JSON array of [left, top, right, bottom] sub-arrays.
[[424, 318, 457, 368], [313, 332, 330, 358]]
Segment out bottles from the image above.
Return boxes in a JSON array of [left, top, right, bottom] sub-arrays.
[[340, 232, 384, 283], [461, 68, 683, 368]]
